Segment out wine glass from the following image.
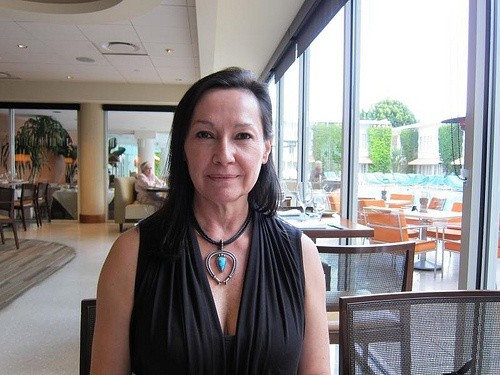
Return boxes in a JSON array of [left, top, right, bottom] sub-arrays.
[[297, 181, 312, 222], [311, 193, 326, 225]]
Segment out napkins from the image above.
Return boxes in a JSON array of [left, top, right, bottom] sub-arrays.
[[276, 207, 300, 217]]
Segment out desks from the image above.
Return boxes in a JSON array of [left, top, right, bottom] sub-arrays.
[[52, 187, 114, 220], [276, 210, 374, 246], [380, 200, 410, 206], [148, 185, 170, 199], [387, 206, 462, 271], [0, 178, 30, 223]]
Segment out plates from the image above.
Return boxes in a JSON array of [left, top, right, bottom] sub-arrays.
[[322, 211, 335, 217]]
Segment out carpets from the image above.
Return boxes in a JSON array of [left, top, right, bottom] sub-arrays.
[[0, 237, 76, 312]]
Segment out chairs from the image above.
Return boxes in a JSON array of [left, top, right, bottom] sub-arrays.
[[274, 186, 500, 375], [113, 175, 156, 232], [0, 182, 50, 250]]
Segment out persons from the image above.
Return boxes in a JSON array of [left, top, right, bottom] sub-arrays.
[[134, 159, 168, 209], [89, 65, 332, 375], [309, 160, 327, 183]]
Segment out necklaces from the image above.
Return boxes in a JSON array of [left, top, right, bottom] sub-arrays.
[[195, 210, 252, 285]]
